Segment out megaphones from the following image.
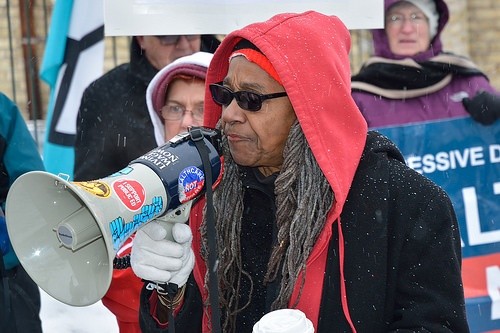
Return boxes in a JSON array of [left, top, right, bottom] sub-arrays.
[[5, 125, 224, 308]]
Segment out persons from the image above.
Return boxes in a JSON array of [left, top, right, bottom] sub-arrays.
[[130, 10, 470, 333], [73, 1, 224, 333], [0, 90, 47, 333], [350, 0, 500, 128]]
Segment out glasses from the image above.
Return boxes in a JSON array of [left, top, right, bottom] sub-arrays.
[[160, 104, 205, 121], [209, 81, 287, 112], [157, 35, 197, 47], [388, 12, 428, 26]]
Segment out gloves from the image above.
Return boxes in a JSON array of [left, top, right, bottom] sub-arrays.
[[130, 219, 195, 289], [461, 89, 500, 125], [252, 308, 314, 333]]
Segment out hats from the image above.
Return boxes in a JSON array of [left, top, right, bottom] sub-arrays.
[[228, 38, 282, 86], [407, 0, 439, 41]]
